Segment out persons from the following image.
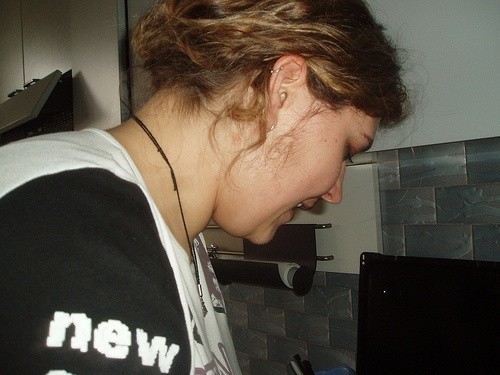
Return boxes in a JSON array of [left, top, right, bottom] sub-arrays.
[[0, 0, 415, 375]]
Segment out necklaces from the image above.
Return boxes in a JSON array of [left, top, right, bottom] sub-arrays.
[[132, 113, 208, 319]]
